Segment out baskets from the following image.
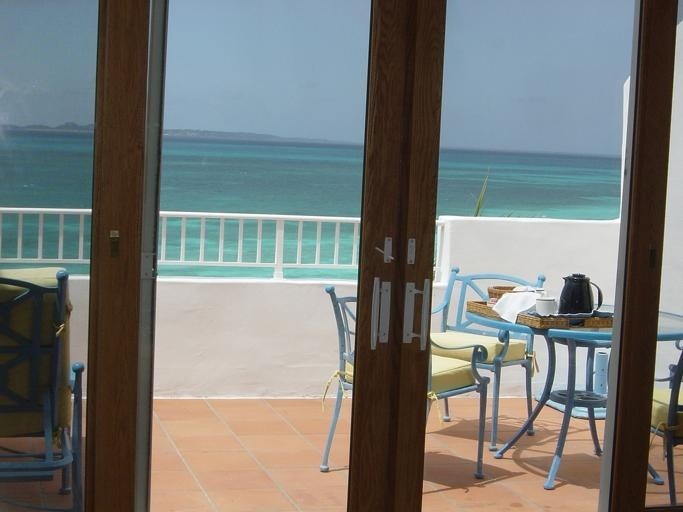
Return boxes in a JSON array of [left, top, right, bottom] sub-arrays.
[[486, 283, 543, 302]]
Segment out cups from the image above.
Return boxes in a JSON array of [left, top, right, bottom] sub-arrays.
[[536, 299, 557, 314]]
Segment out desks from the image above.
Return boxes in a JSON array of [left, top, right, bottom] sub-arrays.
[[465, 302, 683, 490]]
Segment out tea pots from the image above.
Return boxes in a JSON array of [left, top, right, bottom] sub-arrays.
[[558, 273, 602, 314]]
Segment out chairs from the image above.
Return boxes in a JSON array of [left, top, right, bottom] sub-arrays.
[[320, 285, 492, 480], [431, 268, 546, 450], [648, 350, 683, 505], [0, 266, 85, 512]]
